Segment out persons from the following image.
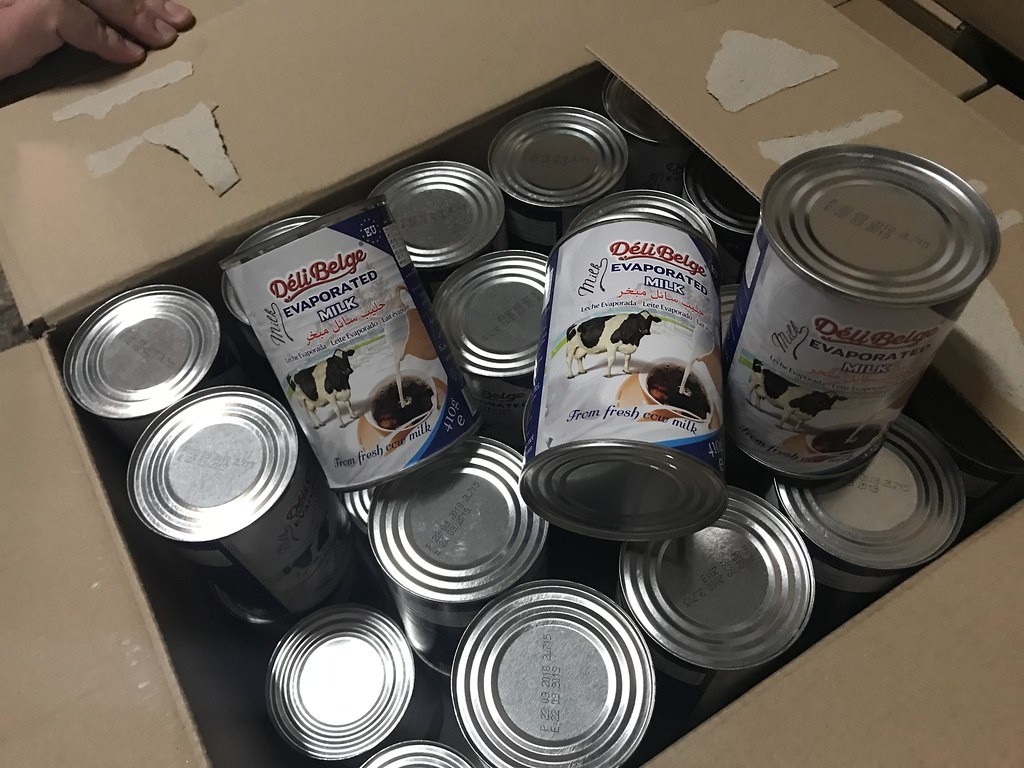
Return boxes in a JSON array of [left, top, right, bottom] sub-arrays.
[[0, 0, 194, 86]]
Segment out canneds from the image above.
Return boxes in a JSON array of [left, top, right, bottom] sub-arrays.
[[61, 100, 1001, 768]]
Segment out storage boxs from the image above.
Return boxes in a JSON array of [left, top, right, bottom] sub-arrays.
[[0, 0, 1024, 768]]
